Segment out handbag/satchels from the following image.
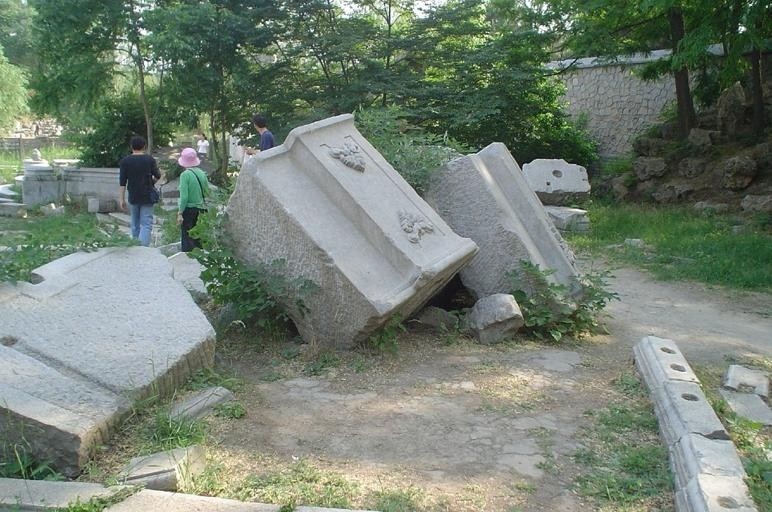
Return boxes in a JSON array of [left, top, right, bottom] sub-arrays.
[[150, 186, 160, 204]]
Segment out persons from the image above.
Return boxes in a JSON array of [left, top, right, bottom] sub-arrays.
[[246, 115, 273, 155], [177, 147, 209, 252], [197, 133, 209, 158], [117, 135, 161, 246]]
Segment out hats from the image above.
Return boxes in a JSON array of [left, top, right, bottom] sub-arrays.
[[178, 148, 200, 168]]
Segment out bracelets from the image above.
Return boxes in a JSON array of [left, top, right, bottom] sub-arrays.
[[177, 211, 183, 215]]
